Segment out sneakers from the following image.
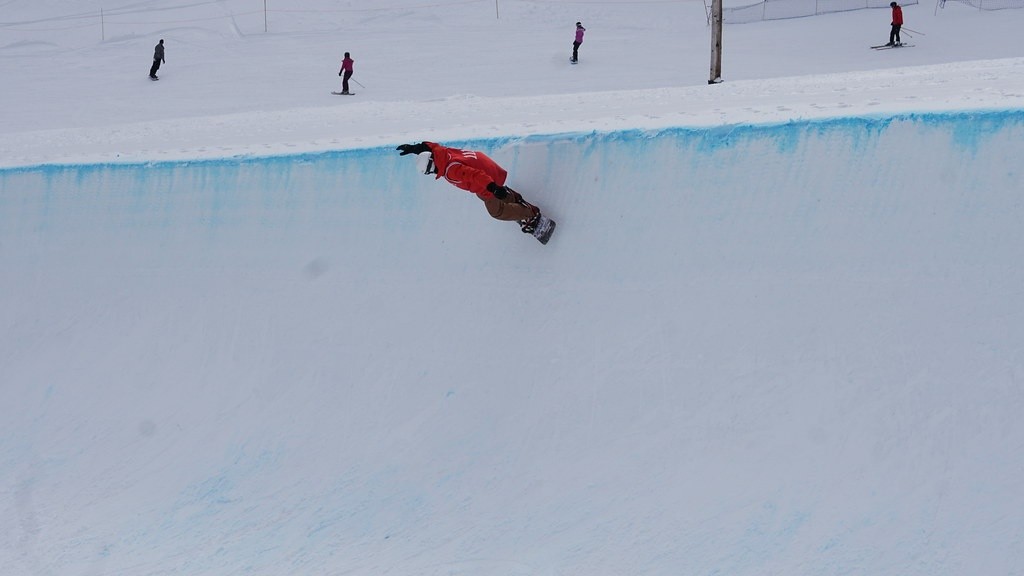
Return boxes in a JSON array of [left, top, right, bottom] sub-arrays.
[[521, 204, 541, 233]]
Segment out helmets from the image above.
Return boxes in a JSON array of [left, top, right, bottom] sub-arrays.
[[416, 151, 435, 177]]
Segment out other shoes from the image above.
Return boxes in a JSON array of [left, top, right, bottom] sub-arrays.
[[150, 73, 158, 78], [341, 91, 348, 94], [571, 59, 578, 62]]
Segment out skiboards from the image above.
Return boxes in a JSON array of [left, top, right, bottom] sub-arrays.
[[331, 91, 356, 95], [871, 41, 916, 51]]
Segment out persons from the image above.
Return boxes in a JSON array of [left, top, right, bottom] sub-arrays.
[[572, 22, 585, 62], [150, 39, 165, 78], [396, 141, 541, 233], [886, 2, 903, 46], [339, 52, 354, 94]]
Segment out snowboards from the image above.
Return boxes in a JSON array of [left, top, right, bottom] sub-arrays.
[[149, 74, 159, 81], [570, 56, 579, 64], [516, 205, 556, 247]]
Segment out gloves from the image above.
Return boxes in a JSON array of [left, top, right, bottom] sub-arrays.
[[487, 182, 513, 202], [397, 144, 426, 157]]
